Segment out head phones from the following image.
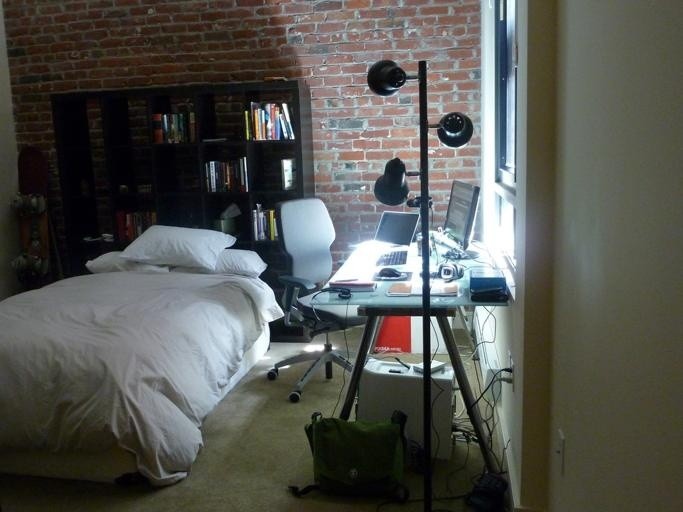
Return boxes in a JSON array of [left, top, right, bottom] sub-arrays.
[[310, 287, 350, 326]]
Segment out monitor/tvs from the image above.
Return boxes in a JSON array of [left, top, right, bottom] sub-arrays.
[[444, 180, 480, 249]]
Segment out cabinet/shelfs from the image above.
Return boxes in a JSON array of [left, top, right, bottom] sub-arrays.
[[50, 78, 316, 280]]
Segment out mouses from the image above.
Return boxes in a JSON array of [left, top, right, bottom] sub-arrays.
[[378, 267, 402, 277]]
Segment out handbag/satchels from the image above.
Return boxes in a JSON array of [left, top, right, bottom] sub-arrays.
[[304, 409, 414, 499]]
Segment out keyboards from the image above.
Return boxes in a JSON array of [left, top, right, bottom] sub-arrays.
[[376, 251, 408, 266]]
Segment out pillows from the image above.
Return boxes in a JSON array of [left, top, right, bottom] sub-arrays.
[[85, 223, 269, 277]]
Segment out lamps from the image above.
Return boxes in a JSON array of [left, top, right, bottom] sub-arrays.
[[366, 59, 474, 512]]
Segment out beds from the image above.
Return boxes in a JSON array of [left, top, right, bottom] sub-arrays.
[[0, 272, 286, 493]]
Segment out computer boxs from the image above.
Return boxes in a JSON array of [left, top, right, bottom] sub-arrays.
[[354, 358, 457, 462]]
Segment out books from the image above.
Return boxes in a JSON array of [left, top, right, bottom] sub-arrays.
[[112, 207, 158, 242], [242, 99, 298, 141], [385, 276, 459, 298], [251, 201, 280, 242], [280, 158, 297, 192], [148, 109, 197, 145], [202, 152, 250, 194]]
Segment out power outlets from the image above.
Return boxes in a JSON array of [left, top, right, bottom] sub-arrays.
[[510, 358, 515, 392]]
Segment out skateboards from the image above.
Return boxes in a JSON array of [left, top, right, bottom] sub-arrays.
[[11, 147, 50, 288]]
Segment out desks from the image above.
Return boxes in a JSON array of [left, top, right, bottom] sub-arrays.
[[310, 235, 509, 476]]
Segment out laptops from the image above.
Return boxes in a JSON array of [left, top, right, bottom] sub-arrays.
[[374, 211, 420, 246]]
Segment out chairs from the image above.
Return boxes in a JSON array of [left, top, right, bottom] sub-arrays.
[[265, 198, 370, 403]]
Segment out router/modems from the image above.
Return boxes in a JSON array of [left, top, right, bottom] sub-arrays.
[[413, 358, 448, 374]]
